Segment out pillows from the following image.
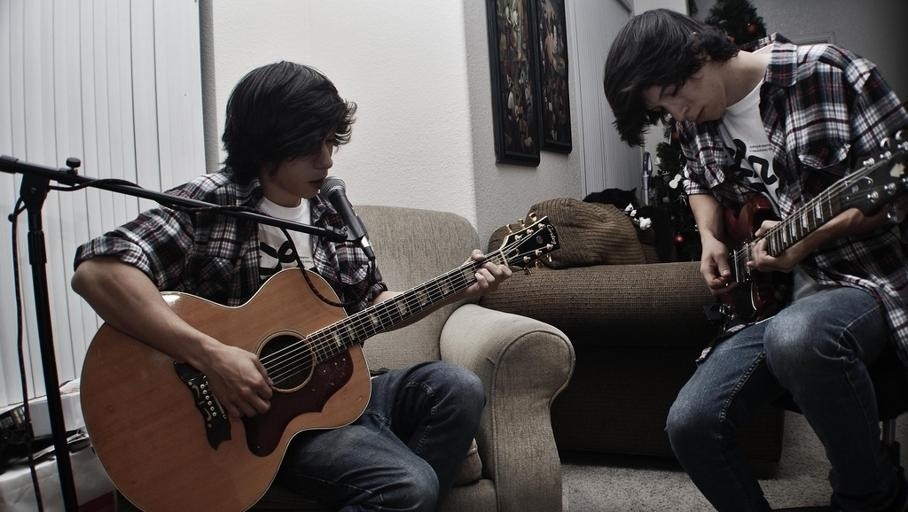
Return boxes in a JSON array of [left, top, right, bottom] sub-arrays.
[[523, 197, 649, 273]]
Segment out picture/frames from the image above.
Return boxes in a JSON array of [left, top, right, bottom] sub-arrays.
[[531, 1, 575, 156], [485, 0, 541, 169]]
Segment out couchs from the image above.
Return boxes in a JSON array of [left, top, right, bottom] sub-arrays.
[[476, 219, 786, 464]]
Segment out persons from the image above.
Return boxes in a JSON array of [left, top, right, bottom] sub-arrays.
[[68, 61, 514, 511], [598, 5, 908, 512]]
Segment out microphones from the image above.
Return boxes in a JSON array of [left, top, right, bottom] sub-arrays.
[[319, 177, 376, 262]]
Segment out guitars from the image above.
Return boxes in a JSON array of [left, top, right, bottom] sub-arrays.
[[716, 130, 907, 325], [80, 212, 561, 510]]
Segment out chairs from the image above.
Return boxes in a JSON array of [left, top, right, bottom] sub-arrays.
[[350, 203, 581, 511]]
